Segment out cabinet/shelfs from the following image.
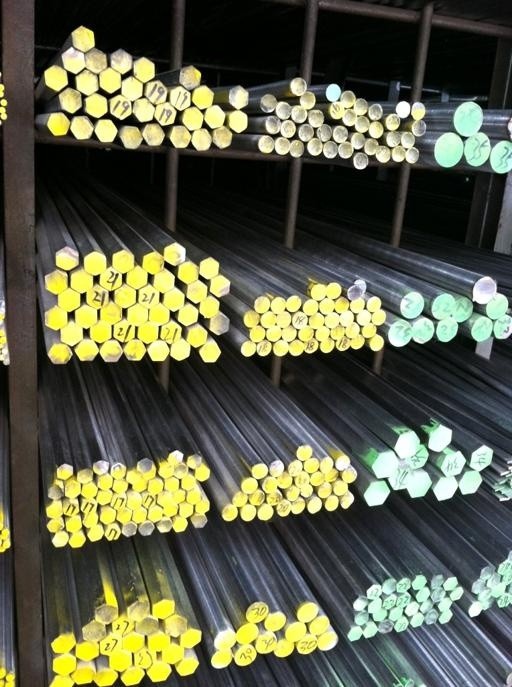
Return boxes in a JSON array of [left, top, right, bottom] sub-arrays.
[[0, 1, 512, 685]]
